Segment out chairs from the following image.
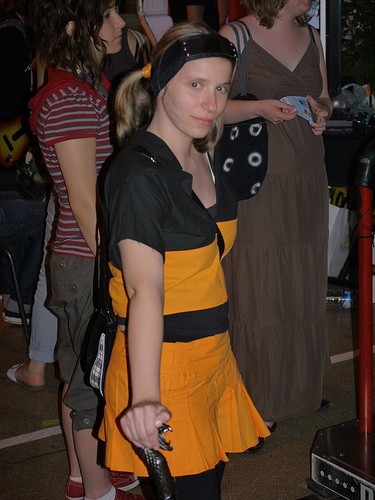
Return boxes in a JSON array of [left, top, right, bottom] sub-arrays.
[[0, 236, 30, 345]]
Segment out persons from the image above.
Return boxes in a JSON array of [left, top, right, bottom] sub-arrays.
[[96, 27, 271, 500], [0, 1, 223, 390], [220, 1, 334, 452], [29, 0, 139, 500], [8, 27, 149, 391]]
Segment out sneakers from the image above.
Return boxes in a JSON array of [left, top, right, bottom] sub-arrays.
[[85, 488, 146, 500], [65, 468, 140, 500]]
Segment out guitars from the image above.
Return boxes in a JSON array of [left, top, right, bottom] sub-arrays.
[[0, 109, 33, 170]]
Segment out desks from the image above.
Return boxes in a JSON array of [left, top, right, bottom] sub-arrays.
[[323, 119, 353, 136]]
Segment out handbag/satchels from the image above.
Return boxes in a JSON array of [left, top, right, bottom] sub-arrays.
[[82, 319, 114, 393], [212, 24, 268, 199]]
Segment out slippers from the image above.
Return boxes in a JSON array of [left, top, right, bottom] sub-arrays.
[[7, 360, 44, 391]]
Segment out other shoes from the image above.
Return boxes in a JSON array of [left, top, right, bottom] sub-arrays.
[[3, 307, 31, 325]]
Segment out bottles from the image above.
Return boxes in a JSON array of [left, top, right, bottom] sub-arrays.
[[325, 288, 351, 310]]
